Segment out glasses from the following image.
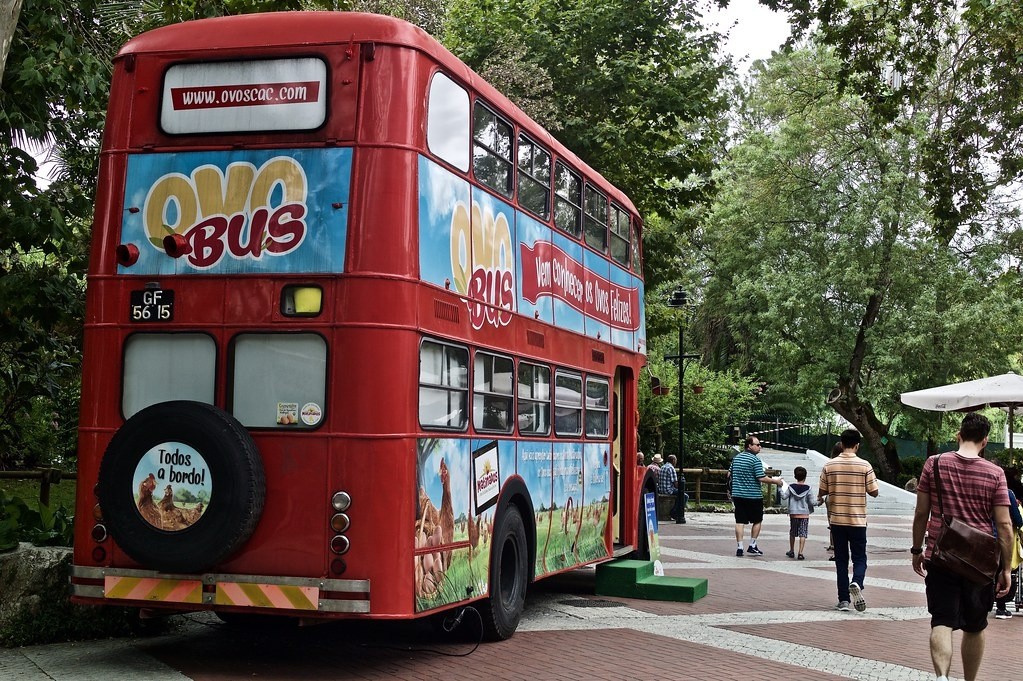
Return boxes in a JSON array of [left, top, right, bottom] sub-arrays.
[[752, 443, 761, 447]]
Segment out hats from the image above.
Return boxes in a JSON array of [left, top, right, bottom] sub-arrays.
[[652, 454, 664, 463]]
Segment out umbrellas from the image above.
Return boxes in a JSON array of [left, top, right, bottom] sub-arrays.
[[901, 370, 1023, 467]]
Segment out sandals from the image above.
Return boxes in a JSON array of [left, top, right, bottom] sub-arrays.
[[786, 551, 797, 558], [797, 553, 804, 560]]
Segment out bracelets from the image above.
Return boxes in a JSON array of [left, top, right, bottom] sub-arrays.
[[910, 546, 923, 554]]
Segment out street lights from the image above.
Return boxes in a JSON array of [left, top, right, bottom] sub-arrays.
[[662, 286, 702, 525]]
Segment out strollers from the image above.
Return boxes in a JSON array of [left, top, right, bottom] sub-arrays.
[[1004, 526, 1023, 612]]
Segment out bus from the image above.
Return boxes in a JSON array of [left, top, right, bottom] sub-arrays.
[[67, 10, 658, 643]]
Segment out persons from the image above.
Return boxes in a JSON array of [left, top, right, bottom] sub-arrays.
[[726, 436, 783, 556], [911, 413, 1013, 681], [658, 455, 689, 520], [777, 466, 824, 560], [644, 454, 663, 494], [827, 442, 844, 561], [818, 430, 878, 612], [994, 489, 1023, 618], [636, 452, 646, 466]]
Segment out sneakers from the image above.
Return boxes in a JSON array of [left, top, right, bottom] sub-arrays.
[[835, 601, 849, 611], [995, 608, 1012, 620], [747, 545, 764, 556], [736, 549, 744, 556], [849, 582, 867, 612]]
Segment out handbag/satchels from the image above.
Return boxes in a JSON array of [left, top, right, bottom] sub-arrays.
[[936, 514, 1001, 586]]
